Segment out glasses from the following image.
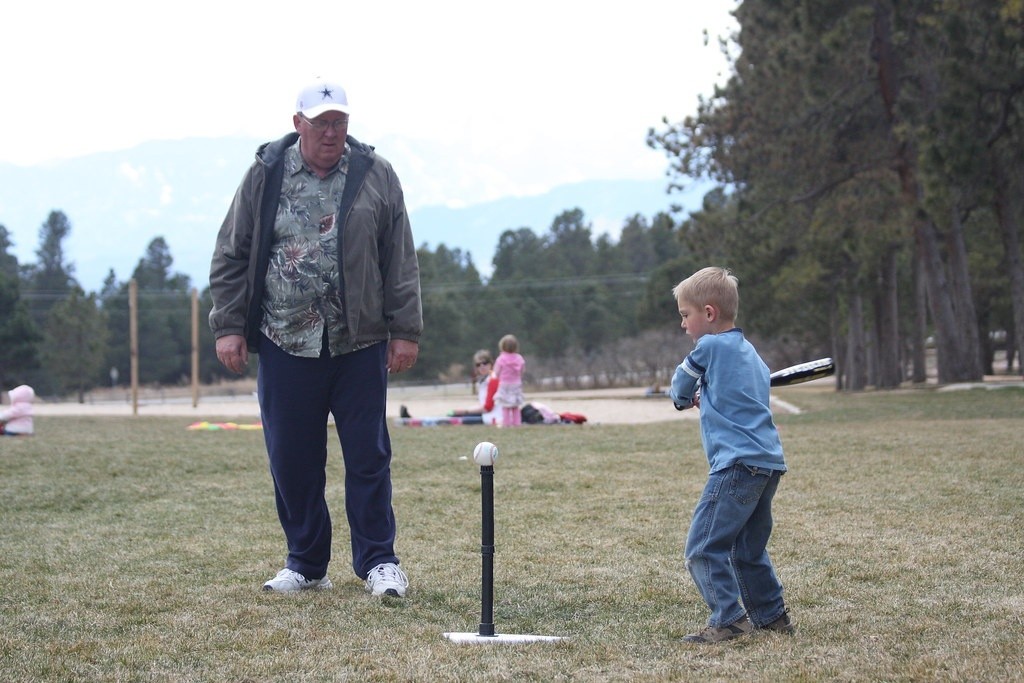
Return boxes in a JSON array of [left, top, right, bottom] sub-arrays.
[[299, 114, 349, 132], [476, 361, 490, 367]]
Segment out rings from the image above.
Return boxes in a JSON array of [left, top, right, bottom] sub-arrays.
[[407, 365, 412, 368]]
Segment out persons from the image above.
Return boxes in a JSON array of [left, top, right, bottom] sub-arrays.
[[668, 267, 796, 647], [491, 335, 525, 428], [0, 385, 35, 436], [210, 78, 424, 599], [400, 349, 502, 428]]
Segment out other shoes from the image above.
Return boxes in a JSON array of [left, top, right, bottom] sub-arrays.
[[401, 406, 410, 418]]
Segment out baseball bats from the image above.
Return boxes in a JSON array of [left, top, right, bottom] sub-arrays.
[[673, 355, 837, 412]]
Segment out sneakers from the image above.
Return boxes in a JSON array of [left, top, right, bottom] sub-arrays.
[[747, 605, 794, 635], [681, 613, 751, 644], [262, 567, 332, 594], [364, 562, 411, 598]]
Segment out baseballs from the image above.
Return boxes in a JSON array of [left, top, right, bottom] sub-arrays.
[[473, 442, 499, 466]]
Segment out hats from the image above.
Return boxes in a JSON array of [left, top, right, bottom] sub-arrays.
[[297, 81, 349, 119]]
